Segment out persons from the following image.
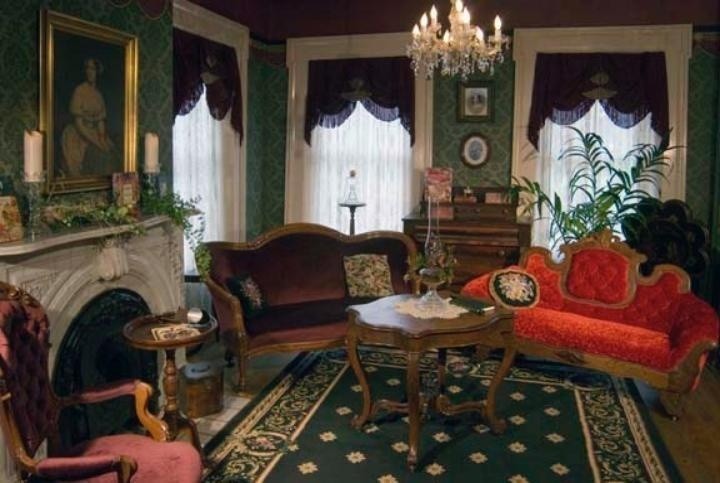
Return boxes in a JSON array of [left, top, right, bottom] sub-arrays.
[[59, 59, 123, 174]]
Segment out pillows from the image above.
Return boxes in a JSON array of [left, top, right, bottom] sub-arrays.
[[224, 275, 269, 320], [343, 253, 395, 298], [489, 269, 541, 312]]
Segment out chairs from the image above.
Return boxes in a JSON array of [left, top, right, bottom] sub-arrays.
[[0, 280, 218, 482]]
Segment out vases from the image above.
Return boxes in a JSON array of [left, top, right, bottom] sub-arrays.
[[403, 245, 458, 311], [348, 182, 357, 201]]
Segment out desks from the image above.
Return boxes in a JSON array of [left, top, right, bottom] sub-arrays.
[[346, 289, 519, 472]]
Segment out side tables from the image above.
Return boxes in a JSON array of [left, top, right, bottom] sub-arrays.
[[121, 307, 220, 465]]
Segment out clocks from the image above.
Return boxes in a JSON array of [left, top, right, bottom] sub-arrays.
[[459, 133, 492, 169]]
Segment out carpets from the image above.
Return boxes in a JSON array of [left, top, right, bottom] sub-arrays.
[[201, 342, 691, 483]]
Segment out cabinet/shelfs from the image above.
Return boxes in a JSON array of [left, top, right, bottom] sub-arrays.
[[401, 185, 532, 295]]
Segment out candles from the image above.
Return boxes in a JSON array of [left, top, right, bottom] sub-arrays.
[[25, 128, 43, 178], [144, 131, 161, 173]]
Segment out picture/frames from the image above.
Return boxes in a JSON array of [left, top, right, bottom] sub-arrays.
[[456, 80, 496, 122], [0, 195, 23, 243], [112, 170, 141, 212], [39, 8, 138, 194]]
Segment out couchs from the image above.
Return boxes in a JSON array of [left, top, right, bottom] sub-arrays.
[[460, 227, 720, 420], [195, 222, 420, 391]]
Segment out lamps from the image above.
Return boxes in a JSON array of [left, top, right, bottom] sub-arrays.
[[406, 0, 511, 79]]
